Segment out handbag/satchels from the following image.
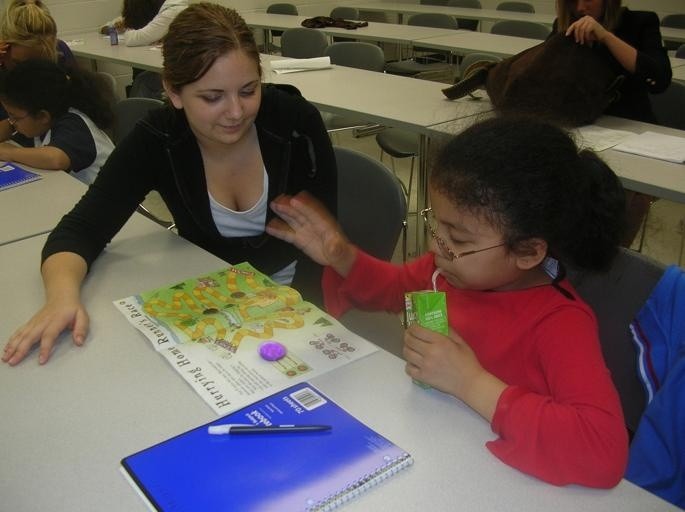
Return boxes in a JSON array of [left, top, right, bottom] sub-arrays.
[[485, 29, 591, 127]]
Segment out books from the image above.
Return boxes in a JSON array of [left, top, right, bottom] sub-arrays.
[[0, 162, 43, 193], [119, 380, 415, 511], [110, 259, 381, 417]]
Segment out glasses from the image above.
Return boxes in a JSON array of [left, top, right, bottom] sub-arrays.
[[420, 208, 505, 263], [7, 113, 26, 125]]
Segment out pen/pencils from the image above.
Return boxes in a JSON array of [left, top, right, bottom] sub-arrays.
[[208, 423, 332, 435]]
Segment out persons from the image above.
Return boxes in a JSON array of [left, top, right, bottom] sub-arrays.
[[1, 1, 116, 186], [100, 0, 191, 103], [0, 4, 338, 367], [267, 111, 630, 490], [546, 1, 673, 251]]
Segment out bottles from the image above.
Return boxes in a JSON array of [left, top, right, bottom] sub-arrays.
[[110, 25, 118, 46]]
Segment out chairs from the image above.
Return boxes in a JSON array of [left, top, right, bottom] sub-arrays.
[[0, 0, 685, 512]]
